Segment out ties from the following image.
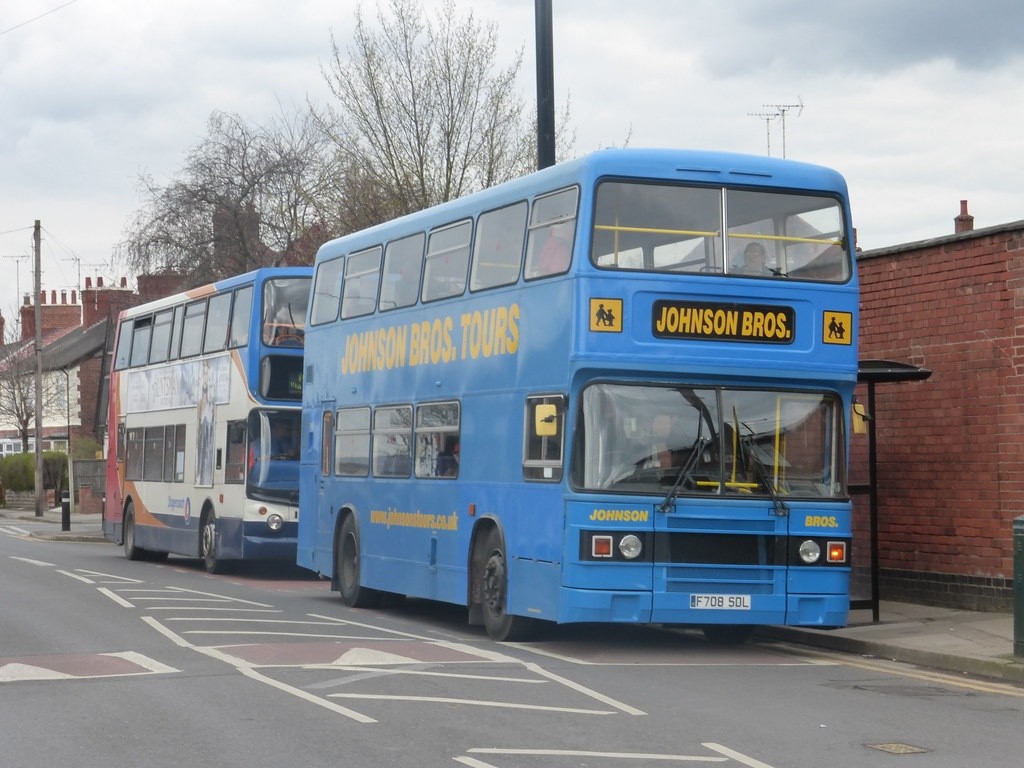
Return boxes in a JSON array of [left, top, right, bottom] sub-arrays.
[[278, 439, 283, 452]]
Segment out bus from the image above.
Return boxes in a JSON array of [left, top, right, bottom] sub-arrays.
[[294, 142, 874, 642], [99, 264, 403, 576]]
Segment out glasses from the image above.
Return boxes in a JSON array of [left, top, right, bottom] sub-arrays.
[[744, 252, 759, 257]]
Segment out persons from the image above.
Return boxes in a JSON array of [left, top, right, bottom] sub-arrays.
[[436, 436, 459, 476], [630, 412, 714, 491], [196, 388, 212, 484], [727, 242, 784, 277], [537, 223, 572, 272], [251, 421, 298, 462]]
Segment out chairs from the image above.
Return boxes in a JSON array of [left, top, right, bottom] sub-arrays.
[[338, 273, 482, 316], [337, 437, 455, 477], [275, 335, 302, 346]]
